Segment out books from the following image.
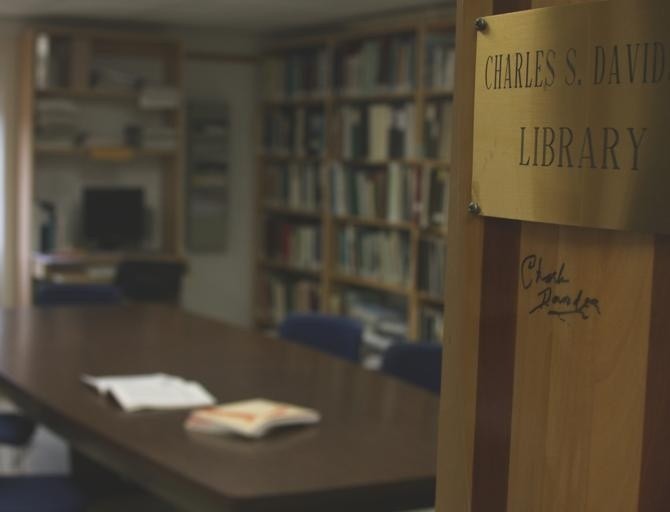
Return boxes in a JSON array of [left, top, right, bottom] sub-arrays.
[[337, 104, 416, 160], [334, 228, 408, 282], [126, 126, 171, 152], [333, 169, 420, 222], [272, 166, 319, 210], [183, 397, 322, 440], [425, 39, 454, 88], [329, 294, 407, 332], [418, 307, 444, 345], [34, 94, 86, 150], [137, 80, 178, 111], [432, 173, 448, 222], [269, 113, 324, 156], [332, 40, 415, 94], [268, 53, 321, 95], [267, 278, 317, 323], [417, 238, 444, 294], [268, 225, 319, 263], [75, 374, 218, 413], [425, 101, 452, 160], [34, 32, 73, 90]]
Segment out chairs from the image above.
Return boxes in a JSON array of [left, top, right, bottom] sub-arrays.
[[90, 260, 187, 300], [0, 473, 83, 512], [380, 343, 442, 398], [0, 412, 37, 468], [274, 317, 362, 363]]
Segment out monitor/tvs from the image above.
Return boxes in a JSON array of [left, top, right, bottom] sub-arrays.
[[82, 186, 145, 242]]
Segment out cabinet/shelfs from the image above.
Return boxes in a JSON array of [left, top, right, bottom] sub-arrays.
[[250, 7, 457, 374], [14, 25, 191, 303]]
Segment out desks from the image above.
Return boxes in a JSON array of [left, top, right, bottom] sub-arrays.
[[0, 300, 440, 512]]
